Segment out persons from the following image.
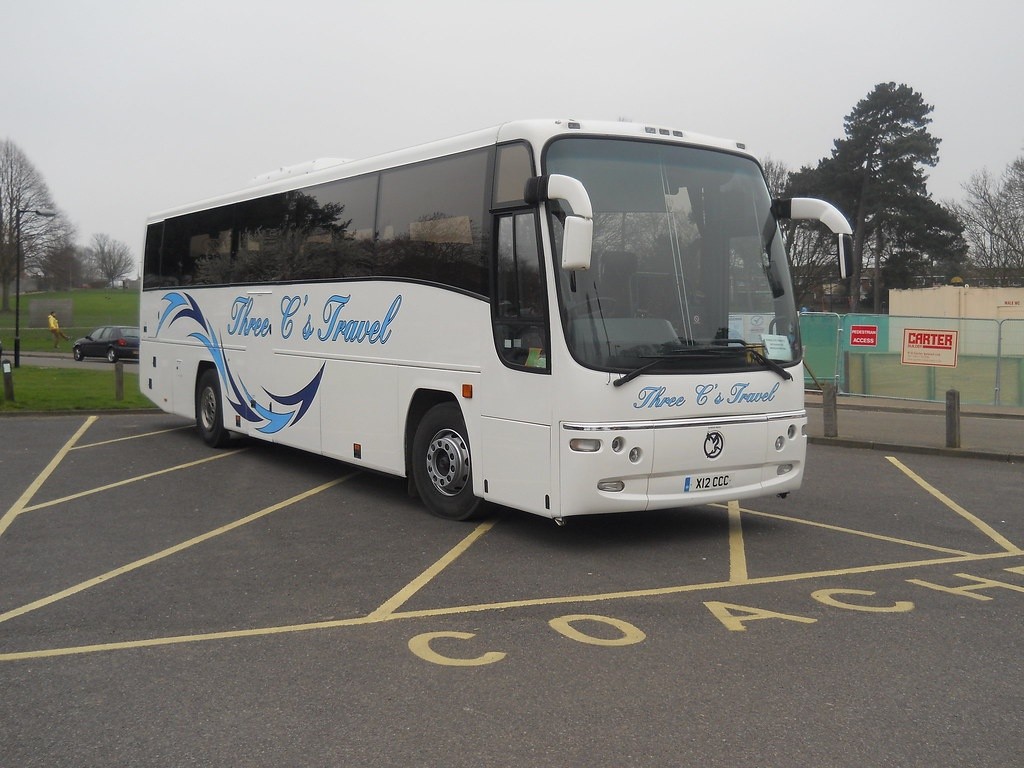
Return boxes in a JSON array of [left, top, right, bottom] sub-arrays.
[[48, 311, 71, 348]]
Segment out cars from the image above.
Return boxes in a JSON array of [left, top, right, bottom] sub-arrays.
[[72, 324, 138, 363], [80, 284, 91, 289]]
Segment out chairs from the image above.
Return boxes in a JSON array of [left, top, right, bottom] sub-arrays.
[[302, 241, 332, 277]]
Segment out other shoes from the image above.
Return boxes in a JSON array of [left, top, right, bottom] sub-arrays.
[[54, 346, 59, 348], [68, 336, 72, 341]]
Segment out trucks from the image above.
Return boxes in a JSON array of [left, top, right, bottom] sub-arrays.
[[105, 281, 124, 289]]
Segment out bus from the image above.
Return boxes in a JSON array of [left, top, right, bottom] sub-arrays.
[[139, 116, 858, 526]]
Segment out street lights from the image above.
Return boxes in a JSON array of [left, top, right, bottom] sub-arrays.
[[13, 207, 57, 367]]
